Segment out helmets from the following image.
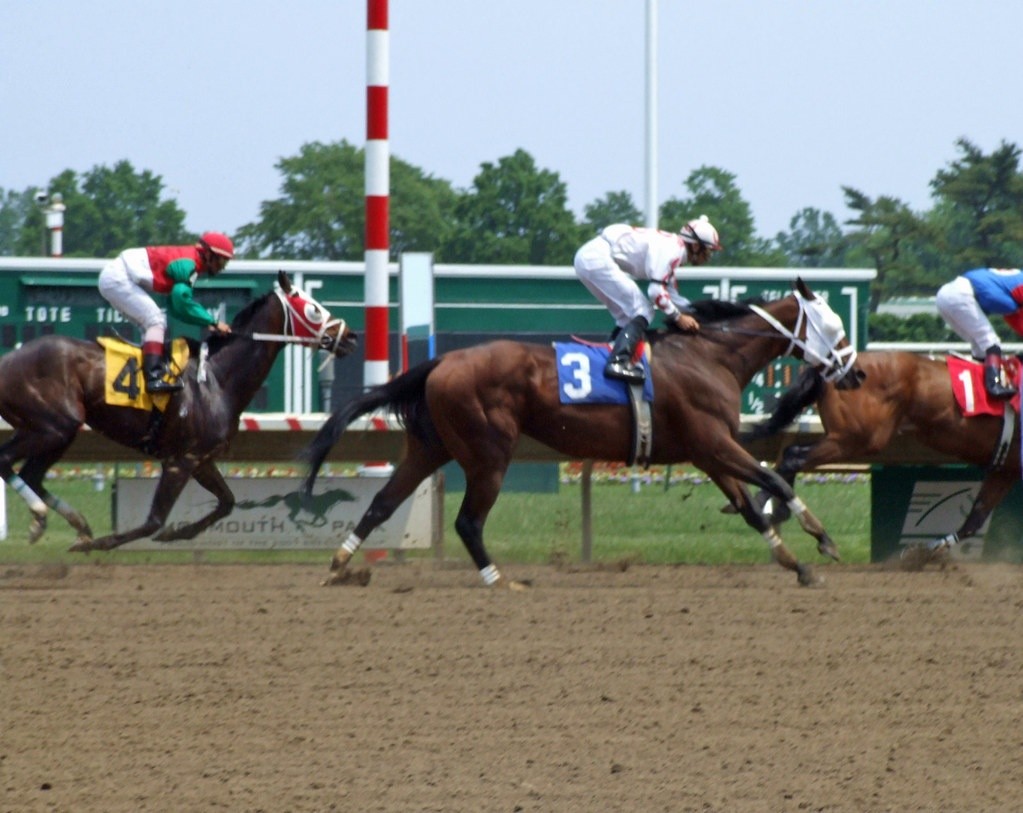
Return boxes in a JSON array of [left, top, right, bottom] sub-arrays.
[[196, 232, 234, 259], [680, 219, 722, 251]]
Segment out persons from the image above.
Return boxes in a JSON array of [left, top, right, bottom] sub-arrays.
[[935, 267, 1021, 399], [97, 232, 237, 394], [574, 214, 720, 382]]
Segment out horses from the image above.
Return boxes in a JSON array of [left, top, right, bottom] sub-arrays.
[[0, 269, 361, 554], [732, 347, 1023, 566], [296, 275, 867, 595]]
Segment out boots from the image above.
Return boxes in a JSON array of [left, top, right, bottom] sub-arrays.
[[142, 353, 183, 392], [986, 346, 1017, 399], [604, 319, 645, 384]]
